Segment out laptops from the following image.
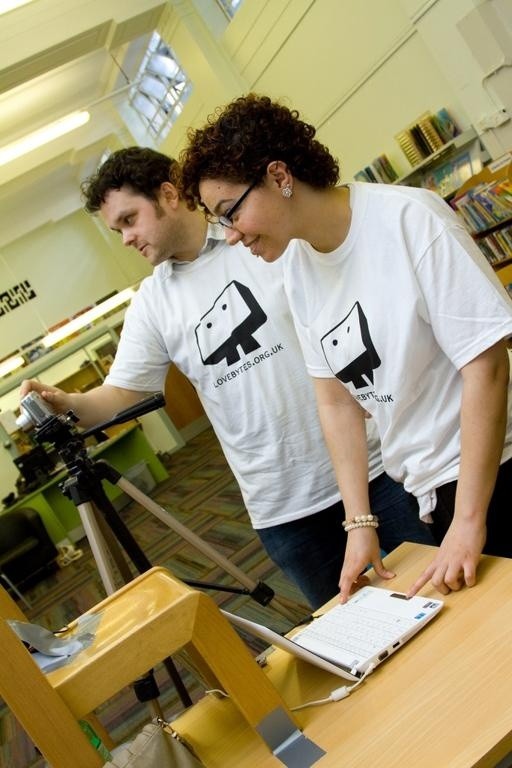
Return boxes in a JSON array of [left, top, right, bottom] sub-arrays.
[[218, 584, 444, 682]]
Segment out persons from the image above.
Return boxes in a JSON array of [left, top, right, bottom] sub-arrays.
[[17, 147, 412, 613], [170, 89, 512, 606]]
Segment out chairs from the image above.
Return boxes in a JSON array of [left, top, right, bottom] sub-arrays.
[[0, 504, 62, 601]]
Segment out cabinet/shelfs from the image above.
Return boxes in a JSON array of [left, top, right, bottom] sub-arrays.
[[370, 125, 511, 298]]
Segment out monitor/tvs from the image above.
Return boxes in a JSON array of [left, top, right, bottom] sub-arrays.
[[13, 445, 54, 483]]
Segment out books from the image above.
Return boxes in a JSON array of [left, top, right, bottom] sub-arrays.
[[353, 107, 511, 300]]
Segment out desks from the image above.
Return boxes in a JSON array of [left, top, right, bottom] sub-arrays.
[[0, 422, 173, 556], [163, 534, 510, 765]]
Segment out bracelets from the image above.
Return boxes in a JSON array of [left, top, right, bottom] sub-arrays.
[[342, 514, 378, 527], [344, 522, 379, 532]]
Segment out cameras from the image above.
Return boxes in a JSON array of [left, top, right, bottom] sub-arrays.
[[15, 390, 56, 432]]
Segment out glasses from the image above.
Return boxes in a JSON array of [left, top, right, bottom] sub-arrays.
[[218, 181, 258, 229]]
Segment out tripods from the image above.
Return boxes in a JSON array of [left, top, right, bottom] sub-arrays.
[[33, 391, 301, 723]]
